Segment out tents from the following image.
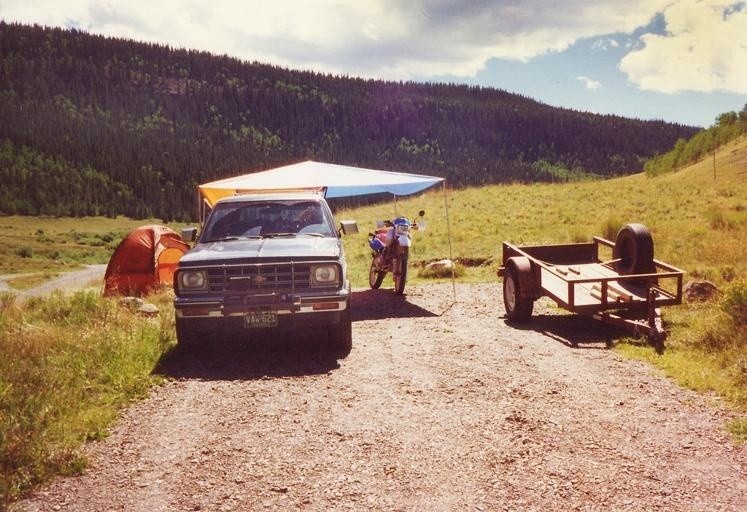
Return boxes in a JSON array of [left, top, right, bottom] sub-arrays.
[[102, 223, 189, 298]]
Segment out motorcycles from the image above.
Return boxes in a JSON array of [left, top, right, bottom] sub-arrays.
[[369, 210, 426, 294]]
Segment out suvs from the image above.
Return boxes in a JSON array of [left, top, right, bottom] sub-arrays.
[[173, 192, 351, 354]]
